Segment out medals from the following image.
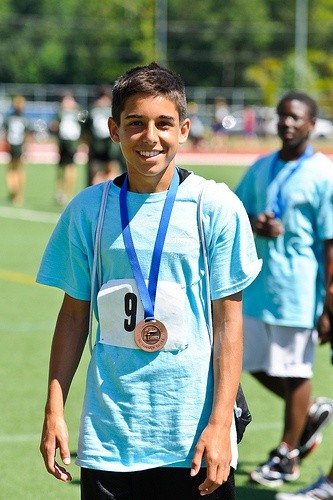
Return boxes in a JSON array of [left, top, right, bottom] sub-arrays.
[[133, 318, 168, 352]]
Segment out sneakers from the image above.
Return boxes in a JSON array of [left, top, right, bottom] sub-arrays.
[[6, 191, 16, 201], [249, 398, 331, 487], [275, 480, 332, 500], [51, 188, 74, 207]]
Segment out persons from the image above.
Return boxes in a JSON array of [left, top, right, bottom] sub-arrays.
[[47, 90, 86, 203], [40, 62, 264, 500], [234, 93, 333, 489], [82, 87, 117, 189], [0, 92, 41, 206]]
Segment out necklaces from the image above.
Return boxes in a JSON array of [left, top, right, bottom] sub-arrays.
[[269, 144, 312, 218]]
